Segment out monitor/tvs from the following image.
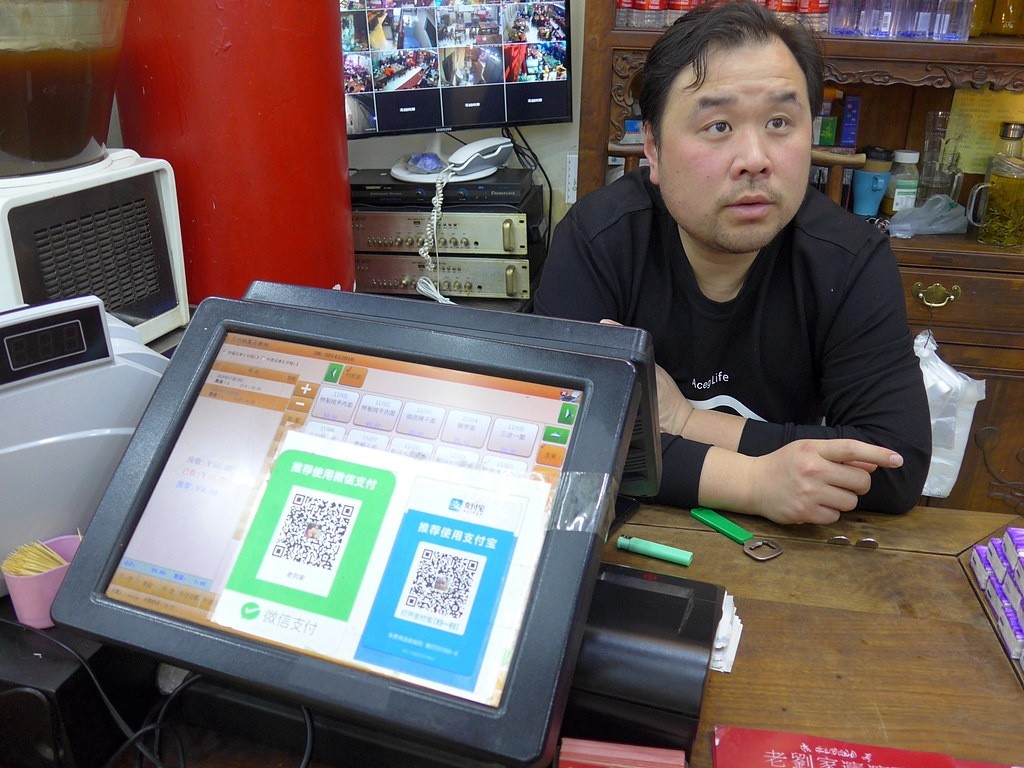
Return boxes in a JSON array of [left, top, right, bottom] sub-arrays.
[[339, 0, 573, 140], [49, 282, 663, 768]]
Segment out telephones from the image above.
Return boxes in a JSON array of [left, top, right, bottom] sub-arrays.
[[448, 136, 513, 176]]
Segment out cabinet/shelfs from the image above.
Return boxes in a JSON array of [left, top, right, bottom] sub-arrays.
[[577, 1, 1023, 515]]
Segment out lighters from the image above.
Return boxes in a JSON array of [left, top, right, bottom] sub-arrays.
[[616, 534, 694, 567]]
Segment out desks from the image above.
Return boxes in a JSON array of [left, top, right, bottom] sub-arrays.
[[123, 495, 1023, 768]]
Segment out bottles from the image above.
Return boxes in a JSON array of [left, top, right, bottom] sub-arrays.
[[613, 0, 1024, 40], [884, 149, 919, 216], [994, 121, 1024, 180]]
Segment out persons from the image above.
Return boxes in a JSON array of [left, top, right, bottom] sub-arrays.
[[339, 0, 568, 94], [534, 0, 931, 529]]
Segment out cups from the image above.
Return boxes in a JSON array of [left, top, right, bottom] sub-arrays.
[[854, 171, 889, 216], [921, 151, 964, 210], [967, 168, 1024, 248], [863, 145, 892, 172], [922, 112, 971, 187]]
